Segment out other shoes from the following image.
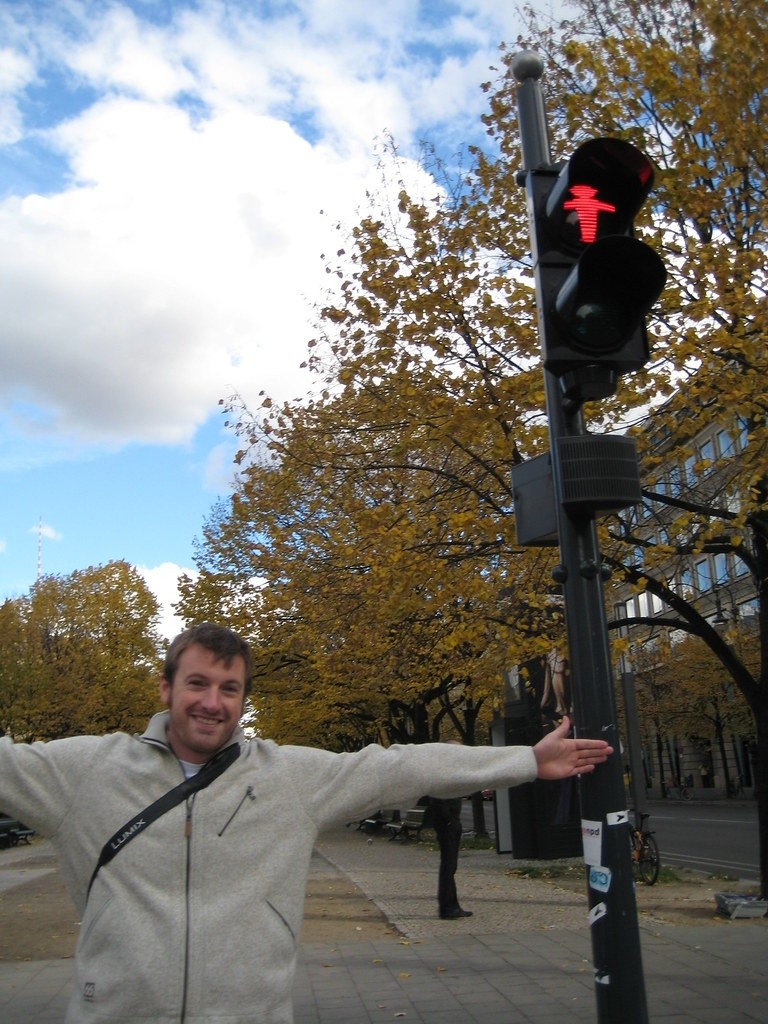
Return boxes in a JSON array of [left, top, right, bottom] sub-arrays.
[[440, 906, 473, 919]]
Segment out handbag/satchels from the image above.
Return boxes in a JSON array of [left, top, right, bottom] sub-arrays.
[[423, 805, 453, 827]]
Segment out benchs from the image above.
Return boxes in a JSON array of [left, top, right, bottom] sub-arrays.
[[346, 809, 401, 838], [385, 805, 431, 844]]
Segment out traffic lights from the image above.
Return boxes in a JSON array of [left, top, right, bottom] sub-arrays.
[[526, 136, 670, 377]]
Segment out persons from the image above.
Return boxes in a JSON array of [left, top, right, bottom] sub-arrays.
[[697, 760, 710, 789], [431, 739, 473, 919], [0, 622, 613, 1024]]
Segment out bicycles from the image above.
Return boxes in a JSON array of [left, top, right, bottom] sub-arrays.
[[665, 775, 695, 801], [623, 806, 661, 885], [730, 774, 743, 800]]
